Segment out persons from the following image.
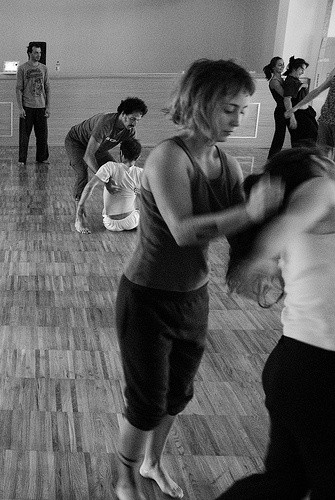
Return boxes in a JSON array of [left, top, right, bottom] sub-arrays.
[[217, 148, 335, 499], [114, 56, 258, 500], [64, 96, 148, 228], [283, 55, 318, 149], [16, 42, 51, 165], [263, 56, 309, 165], [285, 67, 335, 147], [76, 137, 146, 232]]
